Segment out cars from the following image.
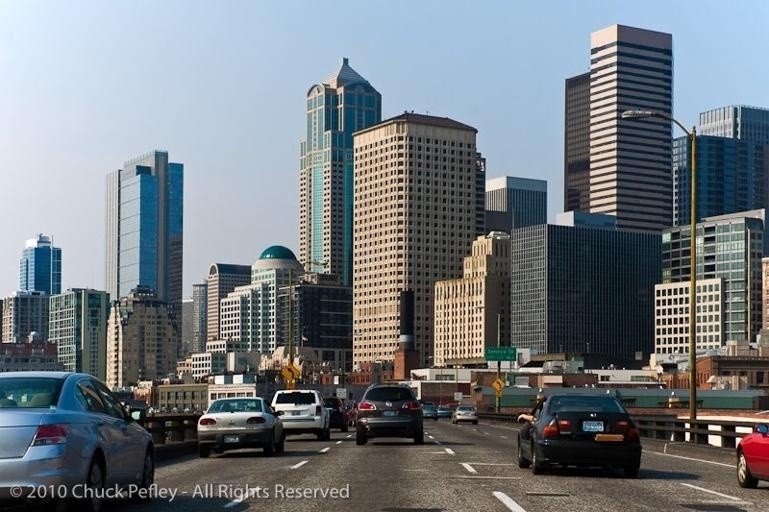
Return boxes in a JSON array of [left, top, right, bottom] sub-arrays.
[[736, 424, 769, 487], [0, 370, 156, 512], [355, 382, 423, 445], [197, 388, 357, 457], [517, 391, 642, 475], [422, 403, 453, 422], [453, 405, 478, 424]]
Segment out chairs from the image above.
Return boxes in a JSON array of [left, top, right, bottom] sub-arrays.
[[222, 401, 232, 412], [0, 392, 52, 407]]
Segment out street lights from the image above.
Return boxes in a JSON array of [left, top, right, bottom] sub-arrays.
[[621, 110, 697, 421]]
